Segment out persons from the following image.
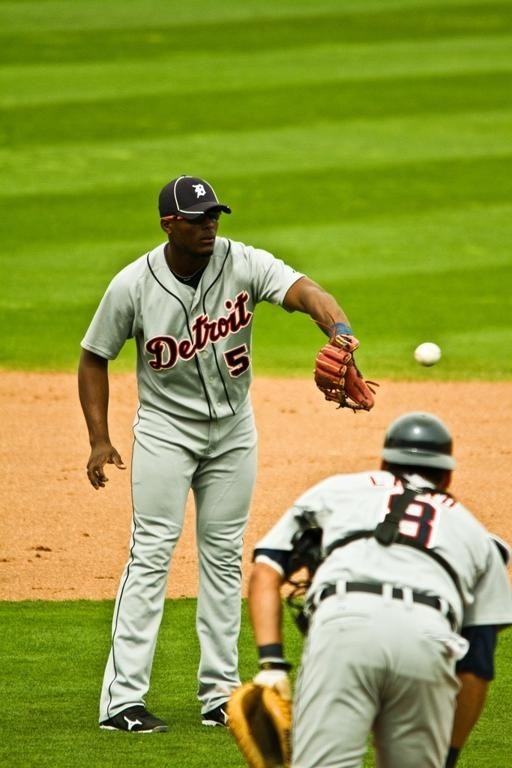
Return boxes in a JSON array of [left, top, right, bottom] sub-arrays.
[[75, 175, 377, 737], [247, 412, 512, 768]]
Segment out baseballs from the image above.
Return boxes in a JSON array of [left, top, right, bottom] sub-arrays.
[[414, 342, 441, 366]]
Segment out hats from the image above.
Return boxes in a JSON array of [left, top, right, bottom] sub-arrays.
[[158, 175, 232, 221]]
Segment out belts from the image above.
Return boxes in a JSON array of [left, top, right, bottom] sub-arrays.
[[309, 581, 456, 634]]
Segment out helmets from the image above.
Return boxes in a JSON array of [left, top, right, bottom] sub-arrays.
[[381, 411, 457, 472]]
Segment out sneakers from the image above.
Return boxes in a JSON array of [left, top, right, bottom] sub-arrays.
[[201, 700, 230, 729], [98, 705, 169, 733]]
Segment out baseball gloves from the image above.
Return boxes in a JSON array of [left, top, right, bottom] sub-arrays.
[[226, 681, 291, 768], [315, 334, 374, 410]]
